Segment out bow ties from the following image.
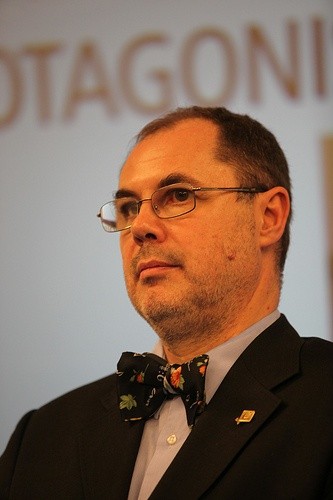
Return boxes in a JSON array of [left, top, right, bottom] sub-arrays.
[[116, 350, 210, 429]]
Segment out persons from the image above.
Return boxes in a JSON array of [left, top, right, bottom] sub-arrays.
[[0, 106, 333, 500]]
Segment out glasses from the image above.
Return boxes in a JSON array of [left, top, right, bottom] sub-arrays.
[[96, 182, 266, 232]]
